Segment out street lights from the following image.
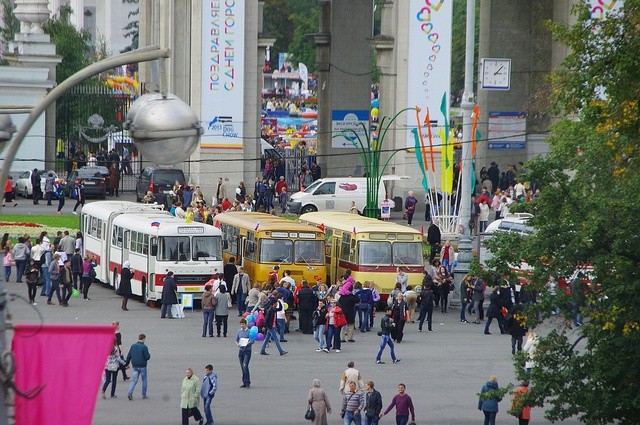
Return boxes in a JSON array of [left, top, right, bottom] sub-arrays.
[[0, 44, 204, 205]]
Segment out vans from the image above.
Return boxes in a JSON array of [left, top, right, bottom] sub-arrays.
[[135, 167, 187, 204], [285, 175, 412, 220]]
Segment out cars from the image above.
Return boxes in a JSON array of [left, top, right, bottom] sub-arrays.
[[15, 169, 60, 200], [66, 169, 107, 200], [81, 165, 112, 196]]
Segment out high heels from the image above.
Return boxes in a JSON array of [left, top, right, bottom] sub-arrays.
[[123, 376, 130, 382]]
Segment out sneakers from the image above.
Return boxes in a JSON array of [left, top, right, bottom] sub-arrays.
[[260, 352, 269, 355], [246, 384, 251, 388], [143, 397, 152, 400], [576, 322, 581, 326], [112, 396, 117, 399], [336, 349, 341, 353], [13, 203, 18, 207], [279, 351, 288, 356], [56, 211, 61, 215], [127, 393, 133, 401], [361, 330, 366, 333], [376, 360, 385, 363], [484, 332, 491, 335], [168, 316, 176, 319], [280, 340, 287, 343], [460, 320, 470, 324], [101, 391, 106, 400], [71, 210, 77, 215], [240, 384, 245, 388], [393, 359, 400, 363], [208, 335, 214, 337], [60, 210, 63, 214], [47, 300, 56, 304], [316, 348, 323, 352], [428, 329, 433, 332], [161, 317, 167, 318], [418, 328, 422, 331], [58, 301, 63, 304], [324, 348, 329, 353], [2, 204, 5, 207], [123, 308, 128, 311], [201, 334, 206, 337], [366, 330, 371, 332]]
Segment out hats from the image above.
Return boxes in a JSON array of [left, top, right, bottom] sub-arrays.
[[490, 376, 497, 381], [330, 298, 337, 303], [395, 292, 404, 300], [53, 254, 61, 258], [123, 260, 130, 267]]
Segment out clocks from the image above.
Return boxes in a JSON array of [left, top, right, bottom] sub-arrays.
[[481, 58, 511, 91]]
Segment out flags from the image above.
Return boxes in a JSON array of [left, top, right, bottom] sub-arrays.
[[401, 92, 483, 197], [151, 221, 161, 227], [318, 222, 324, 231], [13, 322, 122, 425]]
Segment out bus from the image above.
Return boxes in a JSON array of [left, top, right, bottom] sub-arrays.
[[212, 209, 327, 302], [298, 212, 426, 311], [80, 200, 224, 308], [483, 212, 605, 297]]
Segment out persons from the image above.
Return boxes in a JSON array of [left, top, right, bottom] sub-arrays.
[[234, 318, 252, 389], [569, 276, 585, 327], [127, 333, 151, 401], [405, 191, 417, 226], [72, 178, 86, 215], [545, 274, 561, 316], [340, 381, 364, 425], [427, 219, 441, 263], [201, 285, 217, 338], [213, 273, 228, 294], [246, 266, 296, 358], [520, 278, 535, 309], [297, 267, 380, 354], [224, 256, 238, 304], [421, 270, 435, 291], [477, 375, 501, 424], [433, 265, 454, 313], [512, 381, 531, 425], [379, 383, 415, 424], [391, 292, 410, 344], [387, 282, 405, 305], [116, 260, 135, 312], [0, 230, 98, 307], [483, 274, 519, 336], [200, 364, 217, 425], [108, 160, 121, 197], [434, 269, 440, 307], [180, 367, 204, 425], [307, 378, 332, 425], [299, 160, 321, 192], [511, 326, 528, 355], [439, 239, 454, 272], [215, 285, 232, 337], [55, 178, 67, 214], [265, 86, 305, 111], [374, 306, 401, 364], [424, 187, 443, 221], [146, 156, 287, 225], [56, 135, 66, 158], [471, 273, 487, 324], [460, 273, 472, 324], [111, 320, 131, 381], [204, 274, 218, 296], [119, 144, 134, 175], [418, 283, 435, 332], [159, 271, 177, 319], [523, 332, 540, 375], [68, 146, 119, 166], [404, 286, 417, 322], [339, 360, 361, 393], [102, 335, 126, 399], [231, 266, 252, 316], [363, 381, 382, 425], [30, 168, 42, 205], [447, 160, 541, 232], [2, 175, 18, 207], [44, 172, 58, 206], [349, 200, 361, 214], [396, 266, 408, 292]]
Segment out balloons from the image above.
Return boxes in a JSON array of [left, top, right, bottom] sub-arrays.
[[371, 107, 379, 117], [371, 99, 379, 110]]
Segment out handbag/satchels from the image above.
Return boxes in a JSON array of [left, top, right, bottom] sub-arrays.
[[78, 255, 82, 274], [108, 363, 117, 371], [227, 295, 233, 308], [367, 408, 375, 418], [43, 190, 46, 199], [255, 309, 264, 326], [334, 306, 348, 327], [358, 301, 368, 310], [89, 264, 97, 282], [312, 315, 318, 330], [39, 253, 46, 265], [377, 330, 383, 335], [341, 408, 346, 418], [304, 403, 315, 420]]
